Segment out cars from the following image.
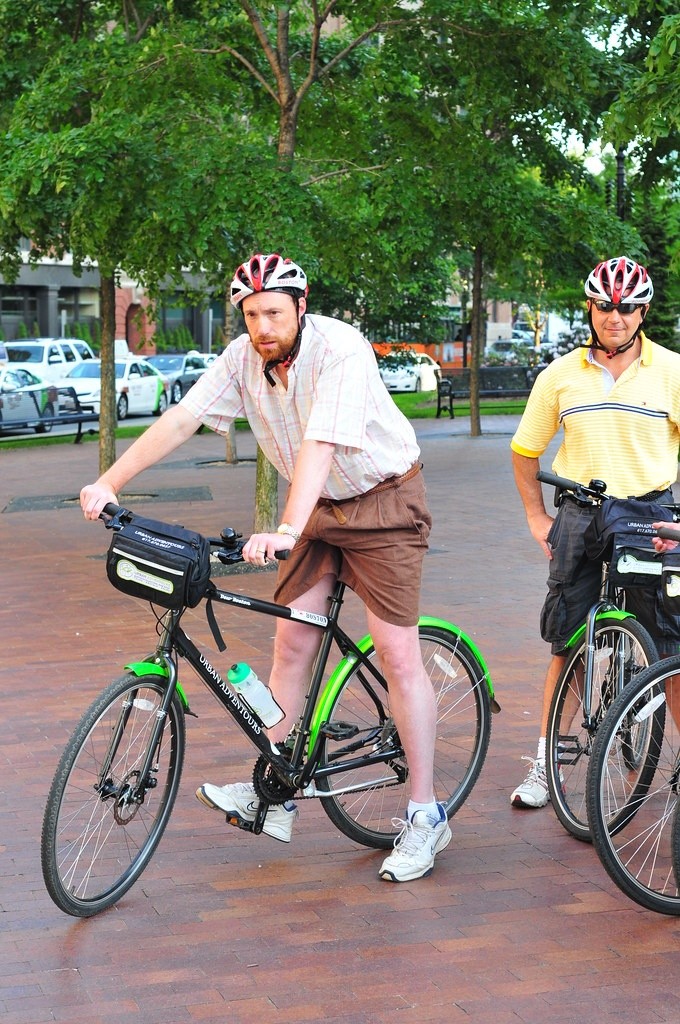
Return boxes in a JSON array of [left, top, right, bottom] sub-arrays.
[[486, 335, 558, 366], [0, 338, 442, 434]]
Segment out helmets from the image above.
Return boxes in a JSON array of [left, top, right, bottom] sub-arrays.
[[584, 256, 654, 304], [229, 253, 308, 310]]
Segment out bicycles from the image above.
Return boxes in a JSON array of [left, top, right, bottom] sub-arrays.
[[535, 467, 680, 844], [585, 656, 680, 920], [40, 497, 500, 920]]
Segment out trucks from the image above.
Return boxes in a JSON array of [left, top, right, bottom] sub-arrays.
[[512, 304, 592, 353]]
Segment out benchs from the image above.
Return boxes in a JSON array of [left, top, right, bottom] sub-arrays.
[[434, 365, 546, 419], [0, 387, 100, 444]]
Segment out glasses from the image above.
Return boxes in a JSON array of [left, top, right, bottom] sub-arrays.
[[591, 299, 643, 314]]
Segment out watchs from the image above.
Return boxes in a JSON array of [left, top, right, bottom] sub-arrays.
[[277, 523, 301, 541]]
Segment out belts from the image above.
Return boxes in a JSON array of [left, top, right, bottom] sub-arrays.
[[635, 485, 671, 503], [318, 459, 421, 525]]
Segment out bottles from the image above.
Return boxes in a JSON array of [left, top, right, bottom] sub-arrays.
[[227, 662, 284, 728]]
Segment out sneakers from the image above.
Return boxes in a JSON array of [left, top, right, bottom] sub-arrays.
[[378, 801, 452, 882], [510, 755, 567, 808], [195, 782, 300, 843]]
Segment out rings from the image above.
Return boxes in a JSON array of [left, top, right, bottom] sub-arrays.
[[256, 549, 265, 553]]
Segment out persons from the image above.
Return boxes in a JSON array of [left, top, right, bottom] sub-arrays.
[[79, 253, 452, 882], [510, 258, 680, 808]]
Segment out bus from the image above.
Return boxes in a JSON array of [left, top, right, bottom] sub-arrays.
[[355, 304, 487, 373]]
